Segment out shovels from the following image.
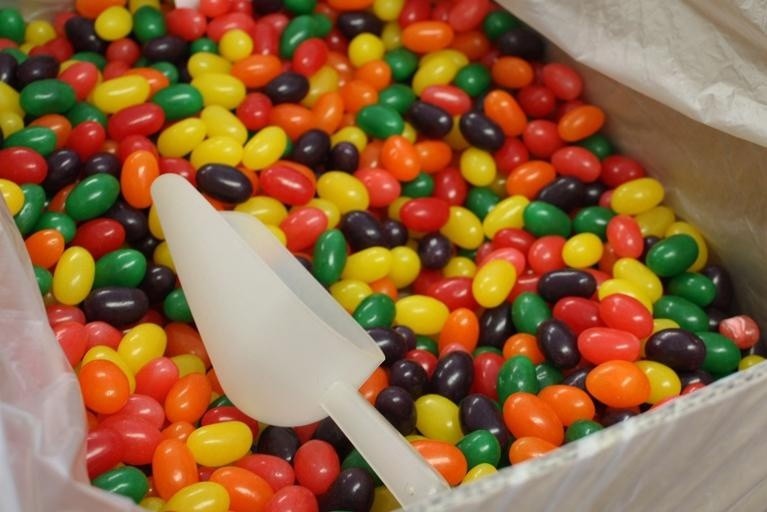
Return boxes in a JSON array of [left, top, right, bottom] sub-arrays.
[[149, 173, 451, 512]]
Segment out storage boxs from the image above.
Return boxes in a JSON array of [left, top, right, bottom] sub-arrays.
[[3, 0, 766, 512]]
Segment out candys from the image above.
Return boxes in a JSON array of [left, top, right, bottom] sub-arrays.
[[0, 0, 766, 512]]
